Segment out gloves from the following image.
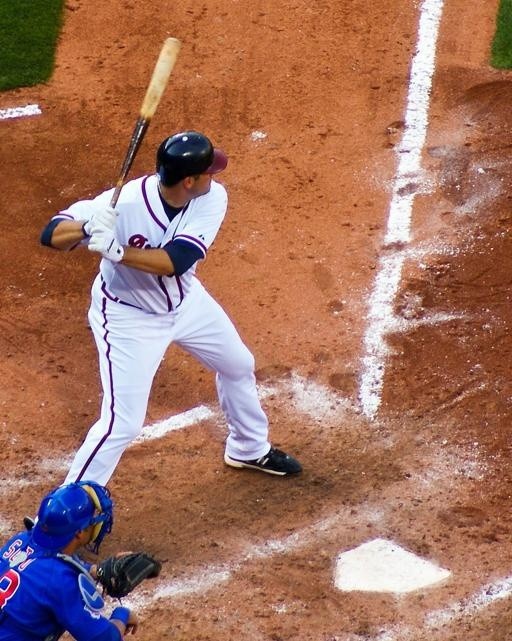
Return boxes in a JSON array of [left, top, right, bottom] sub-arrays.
[[82, 207, 117, 235], [87, 229, 124, 263]]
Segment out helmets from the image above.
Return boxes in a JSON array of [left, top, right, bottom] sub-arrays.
[[156, 130, 229, 186], [31, 481, 98, 552]]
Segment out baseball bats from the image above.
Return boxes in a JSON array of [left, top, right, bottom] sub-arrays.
[[109, 37, 182, 209]]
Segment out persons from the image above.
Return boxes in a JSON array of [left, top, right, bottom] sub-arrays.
[[0, 481, 158, 641], [41, 132, 303, 489]]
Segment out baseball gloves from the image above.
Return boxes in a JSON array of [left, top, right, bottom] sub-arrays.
[[94, 549, 161, 598]]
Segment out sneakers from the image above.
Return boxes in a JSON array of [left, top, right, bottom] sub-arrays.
[[225, 443, 302, 479]]
